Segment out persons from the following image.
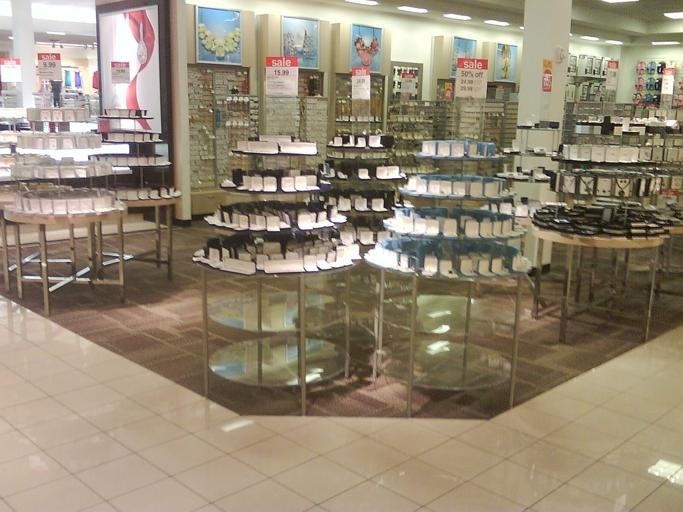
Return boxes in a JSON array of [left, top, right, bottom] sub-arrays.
[[49, 80, 61, 107]]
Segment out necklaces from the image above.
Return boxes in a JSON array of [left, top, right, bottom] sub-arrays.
[[578, 176, 594, 196], [615, 178, 630, 197]]
